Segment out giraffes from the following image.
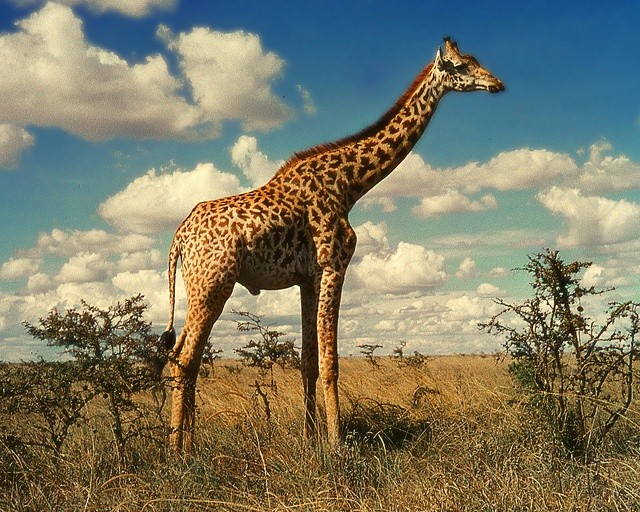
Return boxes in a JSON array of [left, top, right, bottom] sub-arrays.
[[156, 35, 506, 459]]
